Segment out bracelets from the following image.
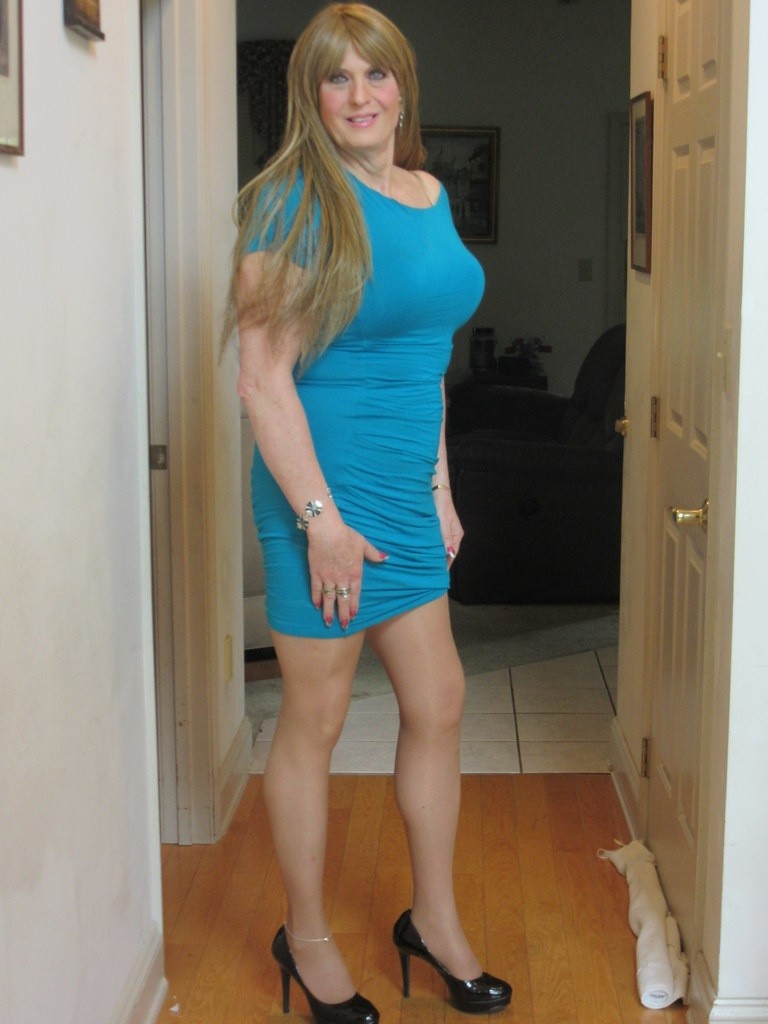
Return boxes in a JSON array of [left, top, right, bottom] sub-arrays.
[[296, 488, 332, 533], [432, 484, 451, 494]]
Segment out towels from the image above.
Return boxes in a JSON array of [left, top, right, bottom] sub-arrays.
[[597, 840, 689, 1008]]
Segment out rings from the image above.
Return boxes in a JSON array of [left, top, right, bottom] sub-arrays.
[[321, 587, 352, 596]]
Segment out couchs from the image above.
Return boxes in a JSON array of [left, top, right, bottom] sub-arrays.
[[448, 323, 626, 605]]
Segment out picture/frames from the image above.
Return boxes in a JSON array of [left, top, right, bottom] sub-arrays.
[[0, 0, 25, 155], [630, 91, 652, 274], [417, 125, 499, 244]]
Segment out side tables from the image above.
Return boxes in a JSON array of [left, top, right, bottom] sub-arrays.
[[463, 367, 547, 391]]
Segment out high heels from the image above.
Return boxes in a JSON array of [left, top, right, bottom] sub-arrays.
[[271, 923, 381, 1024], [393, 908, 512, 1014]]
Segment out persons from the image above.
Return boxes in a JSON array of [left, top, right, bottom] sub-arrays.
[[217, 2, 513, 1024]]
